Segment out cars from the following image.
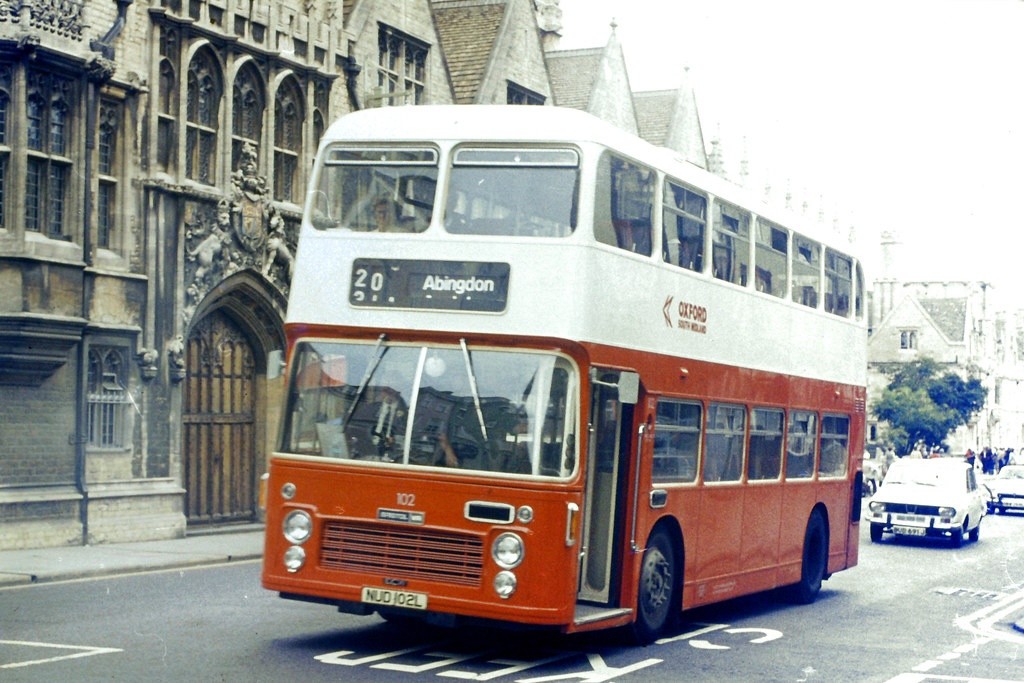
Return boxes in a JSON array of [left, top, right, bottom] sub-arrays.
[[987, 466, 1024, 515], [865, 457, 984, 548]]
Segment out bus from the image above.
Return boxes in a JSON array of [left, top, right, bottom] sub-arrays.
[[260, 105, 869, 644]]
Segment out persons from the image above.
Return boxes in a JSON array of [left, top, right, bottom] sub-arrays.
[[912, 440, 944, 459], [965, 449, 975, 467], [362, 373, 409, 446], [886, 448, 895, 468], [979, 446, 1016, 474], [433, 361, 528, 471]]
